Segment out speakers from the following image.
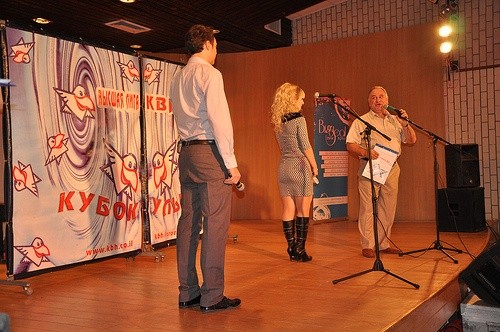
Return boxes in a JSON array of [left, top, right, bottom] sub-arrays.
[[460, 238, 500, 306], [445, 143, 480, 188], [437, 187, 486, 232]]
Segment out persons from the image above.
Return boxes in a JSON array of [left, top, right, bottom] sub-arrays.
[[270, 83, 320, 262], [170, 24, 241, 311], [345, 86, 416, 257]]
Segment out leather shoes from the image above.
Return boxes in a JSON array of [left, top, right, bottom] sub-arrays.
[[379, 247, 400, 254], [200, 296, 242, 314], [361, 249, 375, 258], [179, 295, 201, 308]]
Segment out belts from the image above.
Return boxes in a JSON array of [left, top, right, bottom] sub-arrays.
[[182, 140, 215, 146]]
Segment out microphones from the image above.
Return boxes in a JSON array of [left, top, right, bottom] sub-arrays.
[[228, 173, 245, 191], [384, 104, 401, 113], [314, 92, 336, 98]]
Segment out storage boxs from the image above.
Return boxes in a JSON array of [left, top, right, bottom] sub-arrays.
[[460, 290, 500, 332]]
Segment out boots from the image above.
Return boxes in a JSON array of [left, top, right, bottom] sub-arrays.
[[294, 217, 312, 262], [282, 219, 296, 261]]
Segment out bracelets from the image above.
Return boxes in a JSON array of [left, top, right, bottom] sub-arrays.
[[404, 124, 409, 128], [313, 169, 318, 171]]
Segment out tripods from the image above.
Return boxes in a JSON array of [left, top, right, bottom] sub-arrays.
[[332, 98, 420, 289], [397, 113, 462, 264]]
